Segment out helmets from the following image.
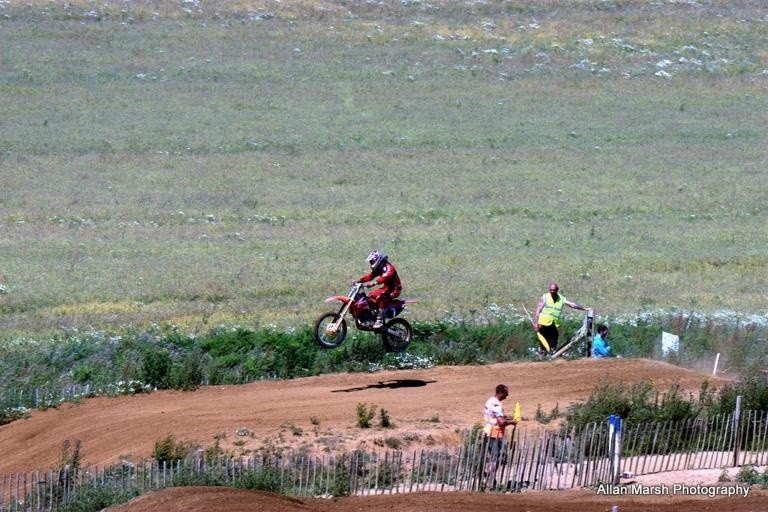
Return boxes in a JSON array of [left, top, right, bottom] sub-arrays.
[[367, 250, 383, 271]]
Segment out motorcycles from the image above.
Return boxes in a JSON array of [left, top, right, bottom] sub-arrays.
[[313, 279, 418, 353]]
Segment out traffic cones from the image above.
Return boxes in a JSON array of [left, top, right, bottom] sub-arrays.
[[512, 401, 523, 423]]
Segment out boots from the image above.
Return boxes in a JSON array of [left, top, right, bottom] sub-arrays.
[[372, 317, 383, 328]]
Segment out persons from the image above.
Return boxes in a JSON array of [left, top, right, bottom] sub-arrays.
[[352, 250, 403, 329], [483, 384, 517, 487], [534, 282, 592, 356], [590, 324, 618, 359]]
